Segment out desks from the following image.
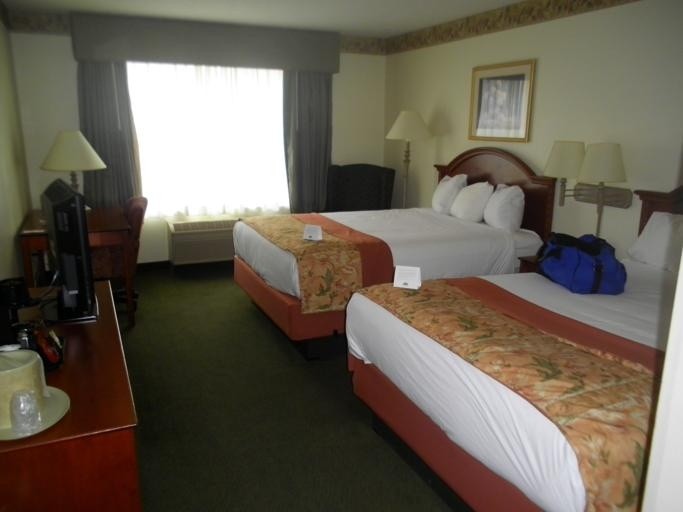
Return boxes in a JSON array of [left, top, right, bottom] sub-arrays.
[[18, 201, 136, 328]]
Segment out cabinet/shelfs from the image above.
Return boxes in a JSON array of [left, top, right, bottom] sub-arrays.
[[0, 279, 144, 511]]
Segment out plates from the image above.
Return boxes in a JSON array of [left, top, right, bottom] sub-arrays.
[[0, 385, 70, 442]]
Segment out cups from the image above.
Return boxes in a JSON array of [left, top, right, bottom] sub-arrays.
[[7, 391, 43, 435], [0, 350, 46, 429]]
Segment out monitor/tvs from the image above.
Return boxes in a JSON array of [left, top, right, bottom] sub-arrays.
[[40, 176, 98, 323]]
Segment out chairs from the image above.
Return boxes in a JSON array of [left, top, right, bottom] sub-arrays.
[[95, 195, 146, 307], [320, 162, 395, 212]]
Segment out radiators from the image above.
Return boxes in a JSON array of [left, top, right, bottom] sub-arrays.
[[167, 217, 233, 266]]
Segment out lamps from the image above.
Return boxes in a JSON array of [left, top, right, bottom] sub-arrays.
[[576, 142, 627, 238], [541, 140, 633, 210], [36, 130, 107, 211], [383, 109, 431, 207]]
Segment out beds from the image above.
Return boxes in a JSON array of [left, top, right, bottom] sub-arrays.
[[346, 184, 683, 510], [231, 147, 556, 370]]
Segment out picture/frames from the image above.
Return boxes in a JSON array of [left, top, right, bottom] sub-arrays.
[[468, 58, 537, 143]]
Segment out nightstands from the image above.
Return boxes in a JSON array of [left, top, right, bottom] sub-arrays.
[[516, 253, 540, 273]]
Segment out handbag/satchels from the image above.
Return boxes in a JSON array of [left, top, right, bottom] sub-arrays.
[[536, 232, 626, 295]]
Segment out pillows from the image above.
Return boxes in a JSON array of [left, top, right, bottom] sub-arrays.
[[429, 173, 467, 215], [483, 183, 526, 235], [626, 208, 682, 277], [449, 180, 493, 225]]
